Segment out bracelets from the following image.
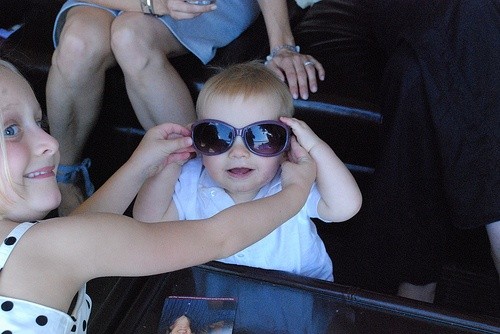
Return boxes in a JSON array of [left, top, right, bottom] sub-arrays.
[[266, 44, 300, 62], [142, 0, 153, 15]]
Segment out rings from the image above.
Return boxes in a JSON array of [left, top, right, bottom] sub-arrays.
[[304, 62, 314, 66]]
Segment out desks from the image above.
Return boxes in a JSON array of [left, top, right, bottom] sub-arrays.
[[84, 260, 500, 334]]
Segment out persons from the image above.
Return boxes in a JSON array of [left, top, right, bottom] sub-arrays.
[[0, 59, 316, 334], [133, 63, 361, 283], [46, 0, 326, 218], [161, 298, 236, 334], [296, 0, 500, 305]]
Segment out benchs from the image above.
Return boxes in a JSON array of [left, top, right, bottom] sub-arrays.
[[1, 1, 403, 178]]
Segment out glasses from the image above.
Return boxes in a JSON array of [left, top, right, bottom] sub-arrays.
[[191, 119, 291, 157]]
